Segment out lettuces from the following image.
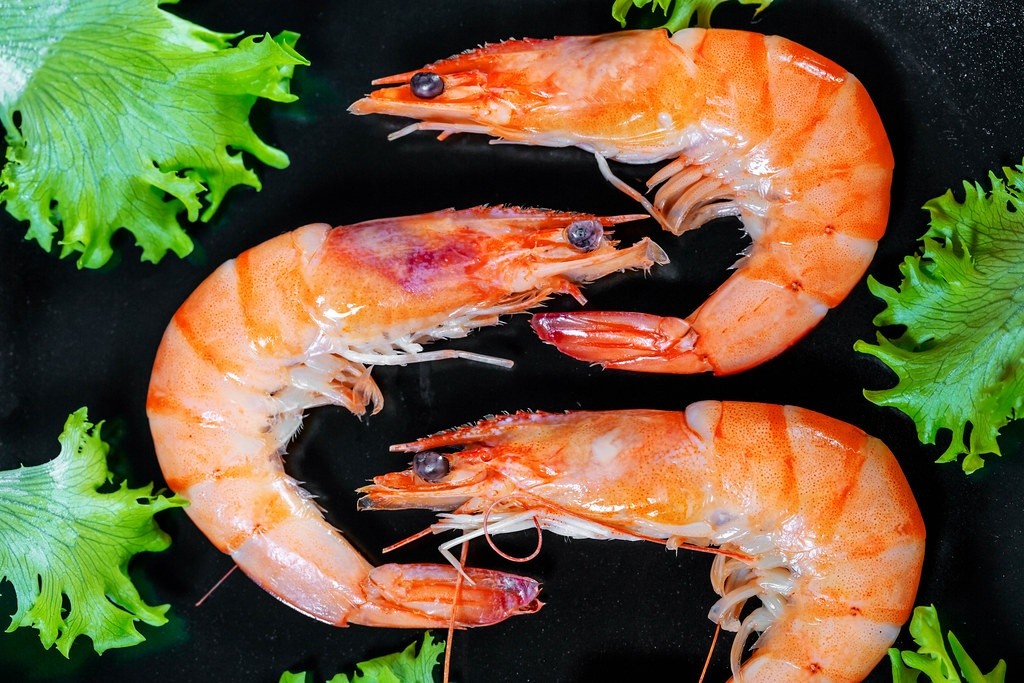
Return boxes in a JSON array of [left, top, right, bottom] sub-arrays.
[[0, 0, 311, 268], [887, 606, 1006, 683], [279, 628, 446, 683], [853, 152, 1024, 475], [0, 405, 191, 657], [611, 0, 775, 36]]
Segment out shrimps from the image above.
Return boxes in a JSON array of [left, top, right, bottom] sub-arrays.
[[146, 27, 927, 683]]
